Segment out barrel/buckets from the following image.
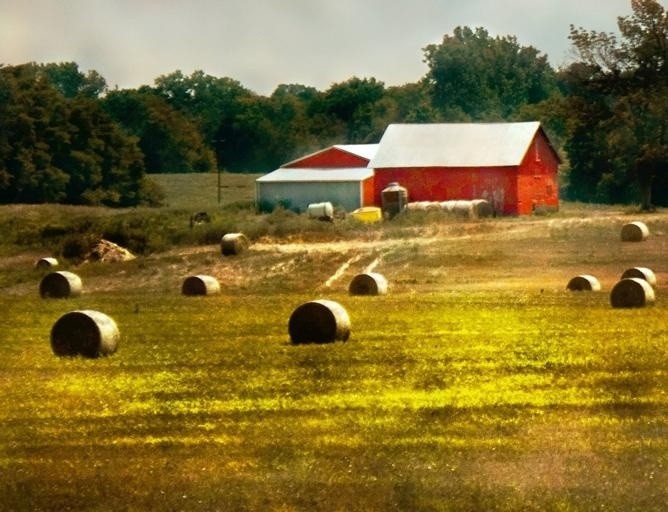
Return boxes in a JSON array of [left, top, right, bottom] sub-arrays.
[[352, 207, 381, 223], [307, 200, 333, 220], [381, 181, 409, 220]]
